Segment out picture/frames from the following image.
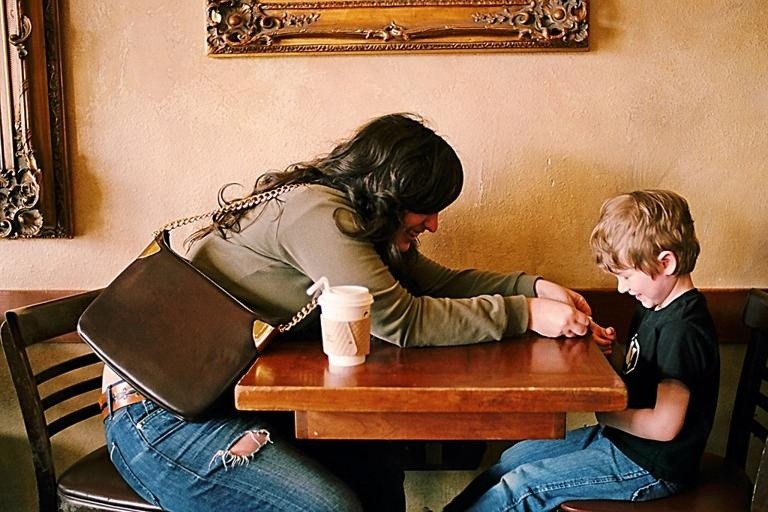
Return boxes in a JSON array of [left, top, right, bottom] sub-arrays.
[[203, 0, 592, 60], [0, 0, 76, 242]]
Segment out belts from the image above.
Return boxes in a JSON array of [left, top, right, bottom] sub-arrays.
[[97, 380, 148, 423]]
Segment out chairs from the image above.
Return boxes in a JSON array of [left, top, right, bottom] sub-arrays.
[[558, 288, 767, 512], [2, 287, 170, 512]]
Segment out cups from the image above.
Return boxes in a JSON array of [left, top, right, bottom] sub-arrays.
[[322, 368, 364, 389], [319, 286, 372, 367]]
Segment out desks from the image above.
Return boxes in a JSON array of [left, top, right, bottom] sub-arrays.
[[233, 331, 630, 442]]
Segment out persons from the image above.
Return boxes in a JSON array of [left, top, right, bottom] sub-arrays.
[[440, 188, 721, 512], [100, 114, 592, 511]]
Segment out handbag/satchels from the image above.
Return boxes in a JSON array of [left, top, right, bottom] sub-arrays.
[[75, 229, 276, 423]]
